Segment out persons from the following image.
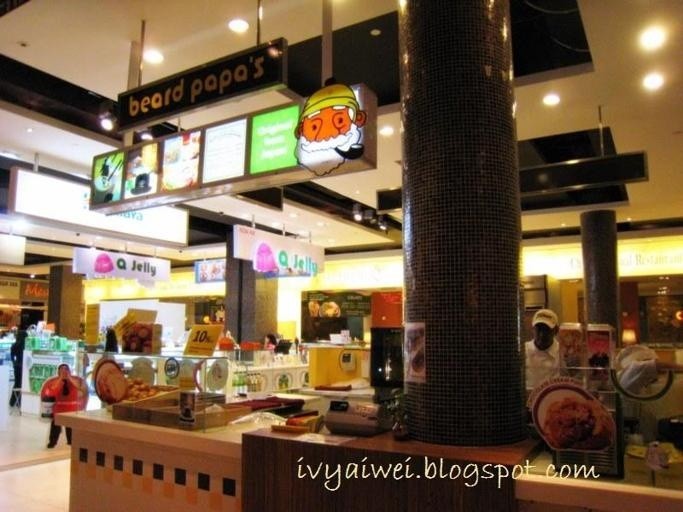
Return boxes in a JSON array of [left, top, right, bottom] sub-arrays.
[[261, 332, 277, 351], [9, 331, 27, 408], [41, 364, 79, 449], [525, 310, 559, 390]]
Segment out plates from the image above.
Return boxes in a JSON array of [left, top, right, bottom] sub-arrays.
[[532, 384, 613, 452]]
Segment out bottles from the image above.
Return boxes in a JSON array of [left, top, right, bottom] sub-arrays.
[[176, 358, 196, 425], [232, 360, 263, 396]]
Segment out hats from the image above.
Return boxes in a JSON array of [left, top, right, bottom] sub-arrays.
[[532, 309, 558, 329]]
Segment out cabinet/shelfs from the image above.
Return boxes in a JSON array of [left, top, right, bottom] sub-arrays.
[[512, 273, 563, 342]]
